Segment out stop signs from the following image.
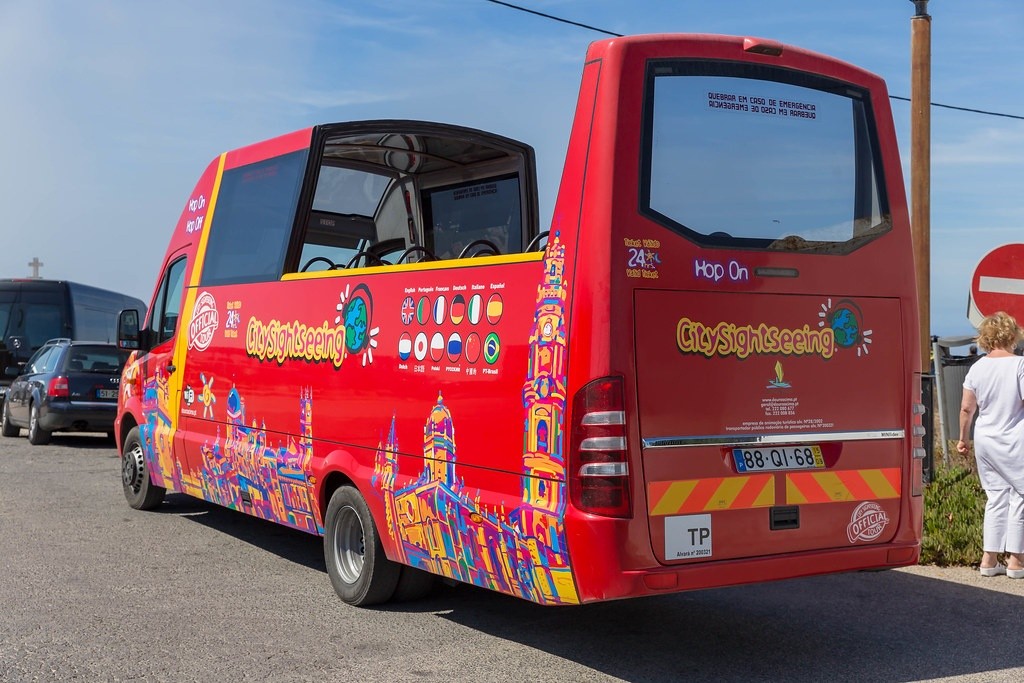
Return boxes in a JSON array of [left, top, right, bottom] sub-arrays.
[[969, 242, 1024, 330]]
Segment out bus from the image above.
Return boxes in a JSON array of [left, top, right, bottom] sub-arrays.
[[115, 32, 926, 608]]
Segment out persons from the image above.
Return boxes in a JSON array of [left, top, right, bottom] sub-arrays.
[[956, 311, 1024, 579]]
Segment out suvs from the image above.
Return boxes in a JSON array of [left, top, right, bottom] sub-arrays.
[[2, 337, 131, 445]]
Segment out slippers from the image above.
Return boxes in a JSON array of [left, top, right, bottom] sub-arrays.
[[1007, 569, 1024, 578], [980, 561, 1006, 577]]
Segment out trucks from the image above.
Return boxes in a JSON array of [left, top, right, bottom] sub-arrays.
[[0, 278, 148, 423]]
